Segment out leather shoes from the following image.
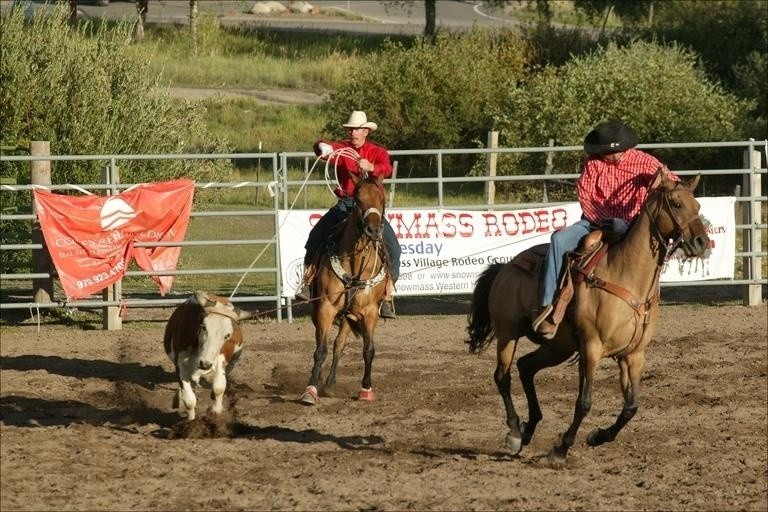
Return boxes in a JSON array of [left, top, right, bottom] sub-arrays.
[[538, 309, 556, 336], [295, 285, 311, 301], [381, 301, 396, 318]]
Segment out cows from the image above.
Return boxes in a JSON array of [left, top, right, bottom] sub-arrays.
[[159, 289, 260, 423]]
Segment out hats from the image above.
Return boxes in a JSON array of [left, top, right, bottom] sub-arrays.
[[584, 122, 639, 155], [341, 110, 378, 133]]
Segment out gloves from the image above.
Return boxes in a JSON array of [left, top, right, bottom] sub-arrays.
[[319, 142, 333, 159]]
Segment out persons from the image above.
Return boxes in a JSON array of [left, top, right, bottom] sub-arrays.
[[534, 121, 682, 343], [293, 108, 401, 320]]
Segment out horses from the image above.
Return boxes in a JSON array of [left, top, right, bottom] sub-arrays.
[[296, 168, 394, 406], [463, 165, 712, 470]]
